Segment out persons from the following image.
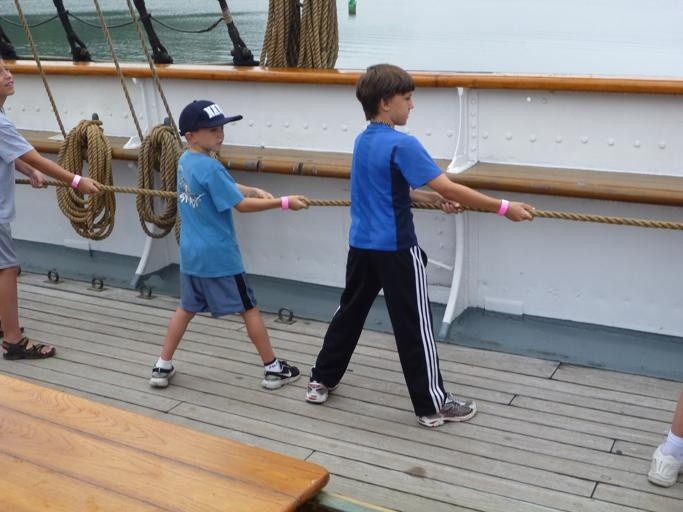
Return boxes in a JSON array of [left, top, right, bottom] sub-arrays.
[[147, 101, 310, 391], [304, 62, 535, 427], [0, 62, 103, 360], [649, 391, 682, 486]]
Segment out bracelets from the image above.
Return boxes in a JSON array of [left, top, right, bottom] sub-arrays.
[[71, 174, 81, 189], [281, 195, 287, 210], [496, 199, 509, 216]]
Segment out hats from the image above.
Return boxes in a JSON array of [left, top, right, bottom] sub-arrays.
[[179, 101, 243, 136]]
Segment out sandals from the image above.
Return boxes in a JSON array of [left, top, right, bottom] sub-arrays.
[[0, 321, 24, 339], [2, 337, 56, 360]]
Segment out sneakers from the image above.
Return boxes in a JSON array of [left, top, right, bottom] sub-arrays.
[[261, 361, 302, 389], [648, 445, 683, 487], [416, 392, 478, 427], [149, 365, 176, 387], [305, 380, 338, 402]]
